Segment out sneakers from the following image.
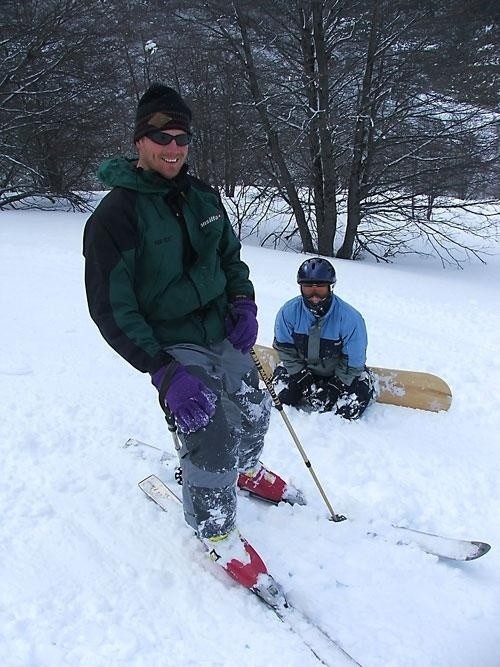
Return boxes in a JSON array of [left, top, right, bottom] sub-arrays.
[[203, 526, 267, 588], [237, 462, 287, 503]]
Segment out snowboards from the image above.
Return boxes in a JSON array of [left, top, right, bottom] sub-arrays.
[[252, 345, 451, 412]]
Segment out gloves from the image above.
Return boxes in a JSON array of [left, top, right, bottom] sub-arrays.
[[152, 360, 217, 434], [291, 368, 348, 413], [226, 296, 258, 355]]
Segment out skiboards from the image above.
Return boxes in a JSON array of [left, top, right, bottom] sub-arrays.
[[120, 437, 490, 666]]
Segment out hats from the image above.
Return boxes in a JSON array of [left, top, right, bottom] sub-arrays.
[[296, 258, 337, 284], [134, 81, 192, 145]]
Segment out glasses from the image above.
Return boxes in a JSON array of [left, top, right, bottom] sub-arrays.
[[146, 132, 191, 146], [301, 285, 328, 298]]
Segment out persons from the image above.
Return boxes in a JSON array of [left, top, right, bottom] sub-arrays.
[[270, 256, 376, 418], [82, 84, 288, 590]]
